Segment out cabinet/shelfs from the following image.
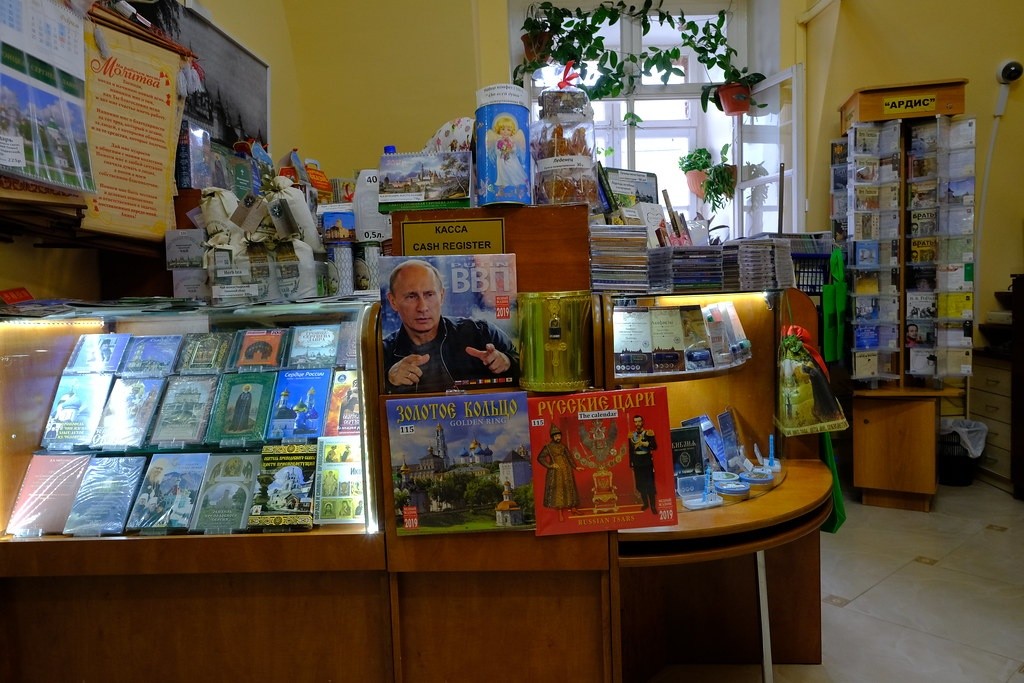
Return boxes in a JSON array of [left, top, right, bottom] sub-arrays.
[[836, 77, 975, 512]]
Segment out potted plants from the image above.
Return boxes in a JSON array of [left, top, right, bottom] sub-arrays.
[[678, 147, 737, 212], [701, 73, 766, 116], [513, 0, 740, 100]]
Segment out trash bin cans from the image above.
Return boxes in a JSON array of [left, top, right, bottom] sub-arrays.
[[937, 420, 989, 487]]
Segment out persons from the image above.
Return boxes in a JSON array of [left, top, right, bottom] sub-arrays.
[[906, 324, 922, 347], [378, 258, 518, 390]]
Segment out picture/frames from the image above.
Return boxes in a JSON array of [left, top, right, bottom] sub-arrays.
[[159, 0, 272, 192]]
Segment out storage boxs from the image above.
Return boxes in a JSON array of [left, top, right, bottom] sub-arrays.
[[386, 391, 537, 537], [164, 229, 207, 270], [174, 269, 212, 301], [526, 385, 678, 537]]
